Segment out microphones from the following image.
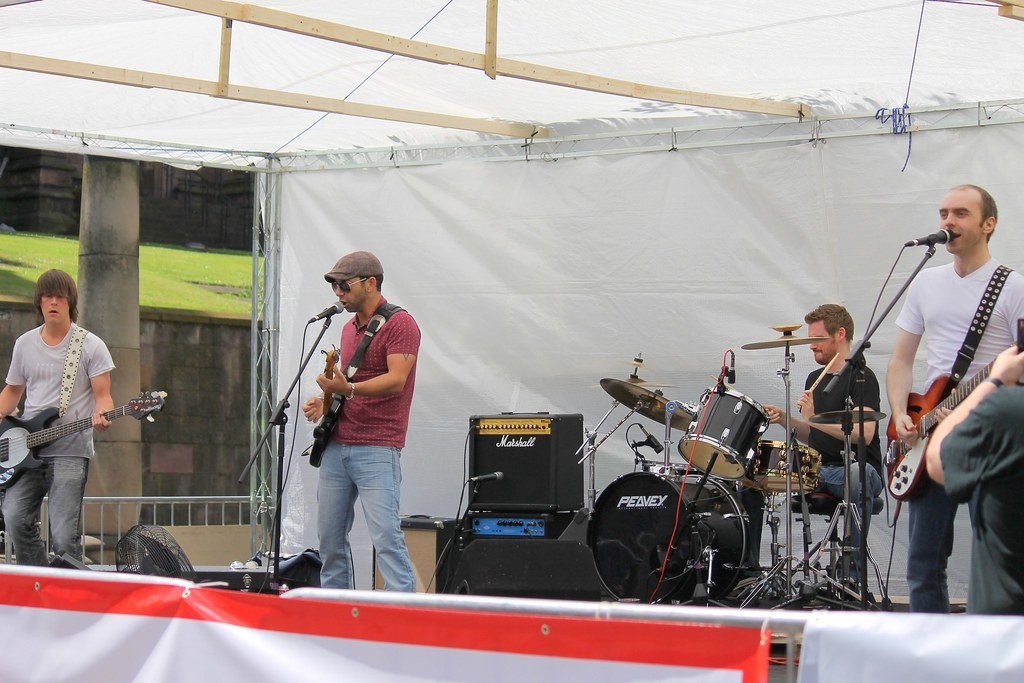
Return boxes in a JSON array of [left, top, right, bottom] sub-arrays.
[[639, 424, 665, 454], [905, 229, 954, 247], [789, 426, 797, 439], [727, 355, 735, 384], [308, 302, 344, 323], [470, 472, 504, 481]]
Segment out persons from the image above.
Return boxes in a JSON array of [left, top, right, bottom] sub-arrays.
[[301, 252, 421, 592], [925, 344, 1024, 616], [0, 269, 117, 569], [887, 184, 1024, 614], [760, 304, 885, 601]]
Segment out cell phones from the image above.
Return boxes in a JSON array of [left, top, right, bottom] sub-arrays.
[[1017, 319, 1024, 354]]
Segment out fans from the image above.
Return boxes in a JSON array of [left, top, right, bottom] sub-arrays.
[[115, 524, 199, 583]]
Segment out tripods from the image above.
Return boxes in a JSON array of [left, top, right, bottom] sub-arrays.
[[678, 342, 894, 610]]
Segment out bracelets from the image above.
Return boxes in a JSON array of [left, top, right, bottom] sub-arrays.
[[981, 377, 1004, 388], [346, 382, 354, 400], [316, 397, 324, 403]]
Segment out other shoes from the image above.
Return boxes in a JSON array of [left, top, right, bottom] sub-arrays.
[[838, 577, 856, 602]]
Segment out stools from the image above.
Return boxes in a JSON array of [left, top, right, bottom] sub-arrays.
[[791, 493, 883, 579]]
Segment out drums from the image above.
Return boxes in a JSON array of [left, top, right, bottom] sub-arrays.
[[742, 439, 821, 492], [589, 470, 747, 607], [677, 387, 771, 481]]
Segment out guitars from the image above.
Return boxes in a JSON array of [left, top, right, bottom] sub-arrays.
[[309, 343, 346, 469], [0, 389, 169, 492], [884, 317, 1024, 502]]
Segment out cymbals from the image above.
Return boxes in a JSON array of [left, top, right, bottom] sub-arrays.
[[591, 379, 679, 390], [741, 323, 832, 350], [808, 410, 887, 425], [598, 377, 694, 432], [621, 359, 648, 368]]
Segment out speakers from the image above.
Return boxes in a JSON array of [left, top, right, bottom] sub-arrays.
[[372, 514, 466, 595], [446, 539, 602, 601], [468, 413, 584, 512]]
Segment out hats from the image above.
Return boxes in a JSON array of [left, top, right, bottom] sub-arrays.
[[323, 250, 384, 283]]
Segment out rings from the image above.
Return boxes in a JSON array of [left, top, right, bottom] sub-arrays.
[[805, 399, 808, 403]]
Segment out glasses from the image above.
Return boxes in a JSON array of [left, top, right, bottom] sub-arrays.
[[332, 278, 367, 292]]
